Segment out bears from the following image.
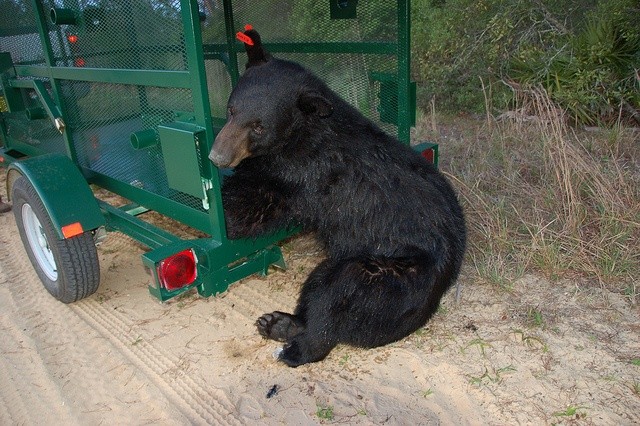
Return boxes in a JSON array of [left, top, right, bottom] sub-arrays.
[[208, 29, 466, 368]]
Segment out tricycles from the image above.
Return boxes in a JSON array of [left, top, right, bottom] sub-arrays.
[[0, 0, 442, 307]]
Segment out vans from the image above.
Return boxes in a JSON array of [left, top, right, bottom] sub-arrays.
[[0, 0, 93, 105]]
